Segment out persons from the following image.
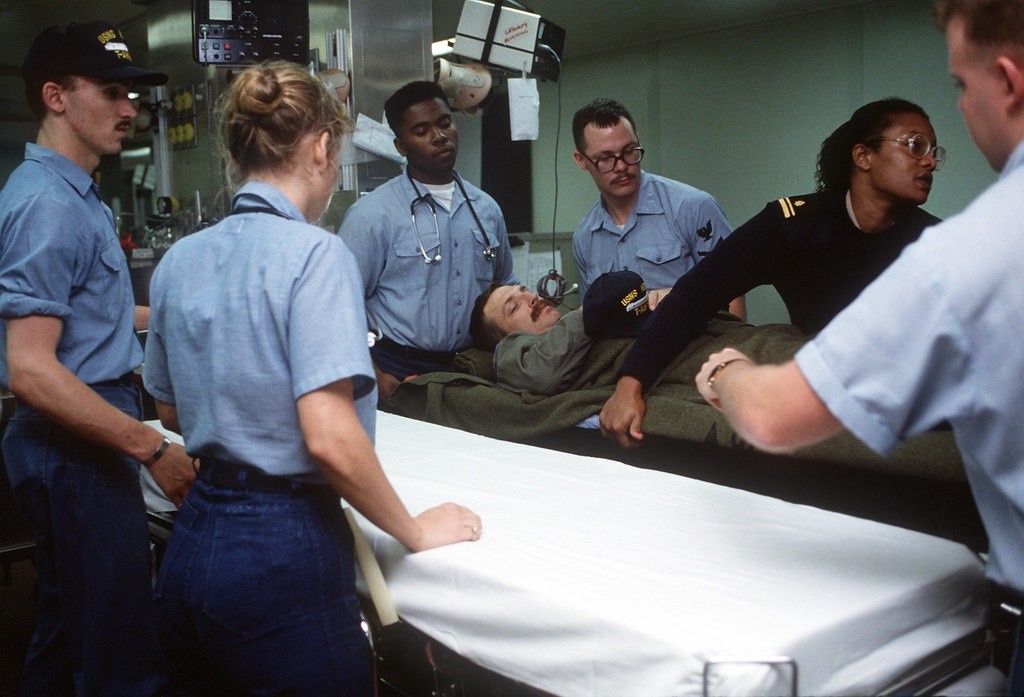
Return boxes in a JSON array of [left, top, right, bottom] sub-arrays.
[[1, 21, 200, 697], [572, 99, 745, 322], [143, 60, 481, 697], [469, 282, 968, 482], [696, 0, 1024, 686], [599, 99, 944, 451], [337, 81, 519, 411]]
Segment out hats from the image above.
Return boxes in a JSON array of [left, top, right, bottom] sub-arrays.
[[21, 19, 169, 89], [583, 268, 654, 339]]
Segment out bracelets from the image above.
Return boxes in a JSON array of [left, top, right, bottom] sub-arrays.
[[144, 439, 171, 465]]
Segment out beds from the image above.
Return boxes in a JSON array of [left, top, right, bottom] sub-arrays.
[[138, 405, 1006, 697]]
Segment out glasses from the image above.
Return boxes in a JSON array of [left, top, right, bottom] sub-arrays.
[[881, 134, 948, 172], [581, 142, 646, 172]]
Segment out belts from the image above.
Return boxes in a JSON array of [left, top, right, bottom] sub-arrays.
[[372, 331, 461, 364], [198, 457, 325, 500], [97, 369, 142, 388]]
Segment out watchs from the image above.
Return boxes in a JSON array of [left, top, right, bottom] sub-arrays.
[[708, 359, 751, 387]]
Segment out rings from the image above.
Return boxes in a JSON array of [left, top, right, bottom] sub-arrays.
[[471, 524, 478, 533]]
